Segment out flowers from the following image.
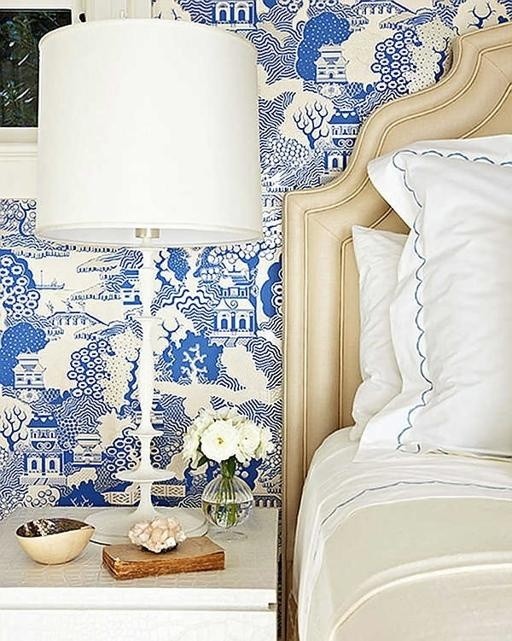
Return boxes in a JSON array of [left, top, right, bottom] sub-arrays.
[[182, 403, 275, 525]]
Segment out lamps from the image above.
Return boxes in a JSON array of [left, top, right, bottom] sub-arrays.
[[34, 17, 262, 547]]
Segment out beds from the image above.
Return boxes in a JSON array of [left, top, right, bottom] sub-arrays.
[[281, 22, 511, 641]]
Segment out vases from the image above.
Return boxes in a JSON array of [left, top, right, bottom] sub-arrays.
[[201, 476, 254, 530]]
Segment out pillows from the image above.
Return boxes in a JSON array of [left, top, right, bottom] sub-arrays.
[[349, 226, 410, 446], [356, 134, 512, 457]]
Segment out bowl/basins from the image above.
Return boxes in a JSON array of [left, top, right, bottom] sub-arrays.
[[15, 517, 95, 565]]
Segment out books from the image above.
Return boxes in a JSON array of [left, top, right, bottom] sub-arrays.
[[102, 536, 224, 581]]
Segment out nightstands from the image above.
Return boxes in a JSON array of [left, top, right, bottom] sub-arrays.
[[0, 506, 279, 641]]
